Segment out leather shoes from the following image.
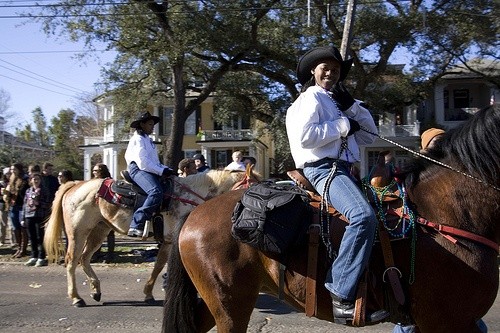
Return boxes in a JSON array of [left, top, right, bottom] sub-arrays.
[[128, 228, 153, 237], [329, 292, 389, 324]]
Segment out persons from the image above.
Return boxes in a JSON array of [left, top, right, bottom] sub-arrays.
[[0, 161, 61, 258], [162, 157, 197, 292], [20, 173, 50, 267], [285, 45, 391, 325], [369, 128, 446, 188], [90, 163, 116, 259], [391, 127, 488, 333], [124, 111, 179, 237], [58, 170, 80, 263], [224, 151, 245, 171], [244, 157, 256, 171], [193, 155, 210, 173]]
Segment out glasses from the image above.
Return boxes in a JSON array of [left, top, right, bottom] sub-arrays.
[[57, 175, 63, 178]]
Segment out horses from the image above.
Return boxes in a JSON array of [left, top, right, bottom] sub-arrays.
[[158, 103, 500, 333], [42, 160, 267, 309]]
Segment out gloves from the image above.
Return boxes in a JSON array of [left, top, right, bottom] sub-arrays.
[[330, 81, 355, 112], [347, 118, 360, 136], [162, 168, 173, 177]]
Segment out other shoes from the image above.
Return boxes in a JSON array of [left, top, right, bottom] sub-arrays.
[[23, 258, 38, 266], [35, 259, 48, 266]]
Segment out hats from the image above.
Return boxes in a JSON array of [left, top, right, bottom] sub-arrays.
[[420, 128, 447, 150], [296, 46, 355, 87], [130, 110, 160, 129], [3, 167, 10, 175], [240, 157, 256, 168], [192, 154, 205, 164]]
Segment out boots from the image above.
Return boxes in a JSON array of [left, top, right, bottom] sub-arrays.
[[15, 229, 28, 258], [12, 230, 21, 257]]
